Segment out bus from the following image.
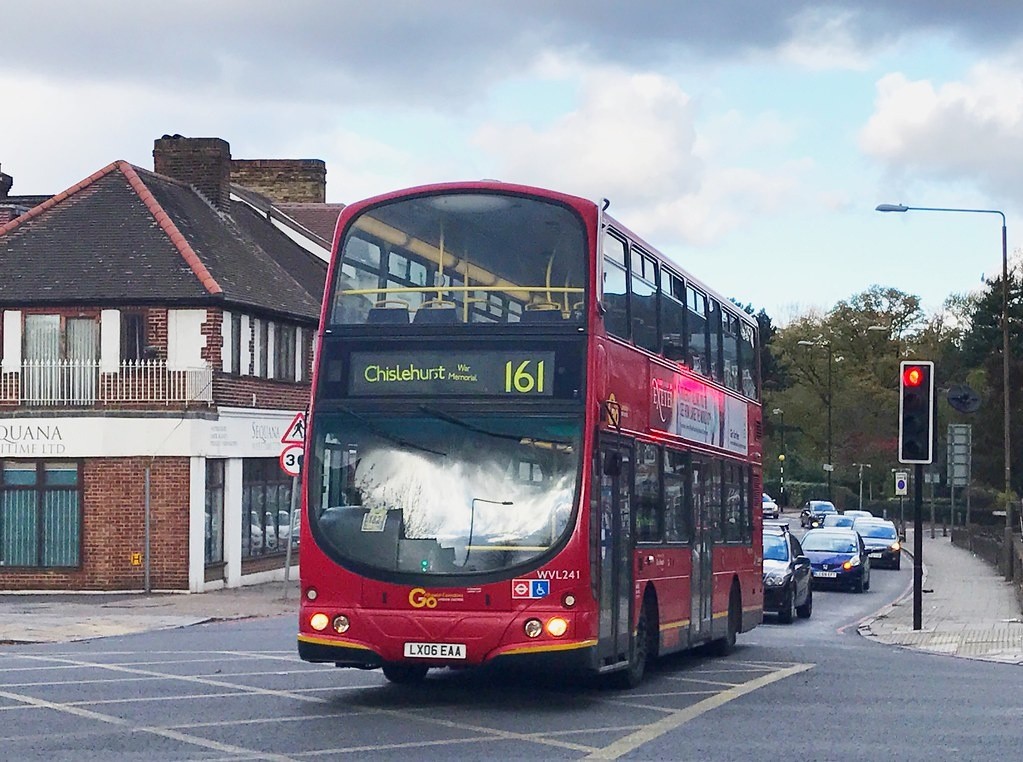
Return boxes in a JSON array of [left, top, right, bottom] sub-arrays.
[[296, 178, 767, 691]]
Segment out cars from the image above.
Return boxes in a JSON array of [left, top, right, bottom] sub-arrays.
[[819, 510, 901, 570], [763, 493, 779, 520], [799, 500, 838, 530], [761, 529, 814, 624], [800, 528, 873, 594]]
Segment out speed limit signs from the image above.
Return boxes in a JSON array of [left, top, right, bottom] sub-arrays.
[[280, 445, 305, 477]]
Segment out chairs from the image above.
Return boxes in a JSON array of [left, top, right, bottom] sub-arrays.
[[412, 301, 457, 324], [366, 299, 410, 323], [520, 302, 563, 321], [765, 546, 786, 561], [570, 302, 699, 373]]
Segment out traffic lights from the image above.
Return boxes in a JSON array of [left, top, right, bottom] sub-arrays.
[[899, 359, 933, 465]]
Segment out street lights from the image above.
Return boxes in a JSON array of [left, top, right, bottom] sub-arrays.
[[772, 408, 785, 515], [891, 468, 911, 535], [875, 204, 1014, 585], [853, 463, 872, 510], [798, 339, 832, 503]]
